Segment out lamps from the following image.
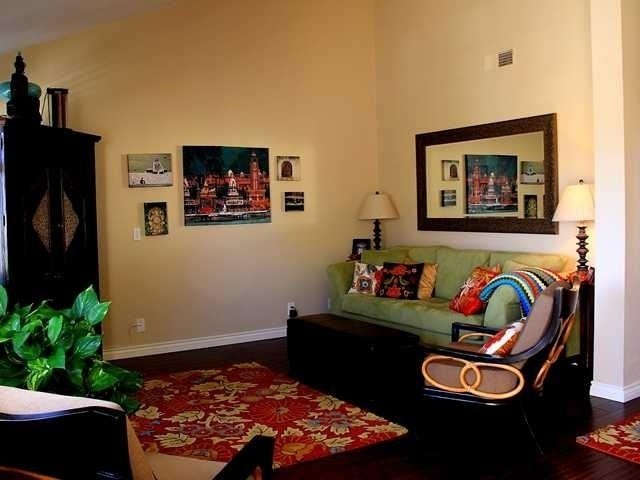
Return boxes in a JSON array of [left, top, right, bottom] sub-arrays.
[[357, 187, 401, 250], [549, 179, 596, 281]]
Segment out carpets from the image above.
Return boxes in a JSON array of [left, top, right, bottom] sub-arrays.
[[568, 406, 640, 471], [121, 348, 417, 477]]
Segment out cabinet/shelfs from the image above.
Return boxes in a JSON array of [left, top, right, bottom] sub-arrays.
[[0, 115, 104, 361]]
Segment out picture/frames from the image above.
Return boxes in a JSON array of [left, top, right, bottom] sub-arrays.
[[351, 235, 372, 261]]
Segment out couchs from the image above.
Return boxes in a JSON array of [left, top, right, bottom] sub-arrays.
[[316, 242, 587, 366]]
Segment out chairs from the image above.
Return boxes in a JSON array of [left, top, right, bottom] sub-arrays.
[[0, 381, 277, 478], [418, 273, 587, 461]]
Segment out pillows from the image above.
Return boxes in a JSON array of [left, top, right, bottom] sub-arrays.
[[447, 263, 503, 316], [475, 316, 528, 356], [416, 262, 439, 301], [376, 259, 426, 302], [347, 260, 386, 298]]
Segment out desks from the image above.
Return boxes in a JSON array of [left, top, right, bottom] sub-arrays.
[[282, 311, 424, 398]]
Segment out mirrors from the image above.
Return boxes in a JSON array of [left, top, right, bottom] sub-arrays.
[[411, 112, 564, 237]]
